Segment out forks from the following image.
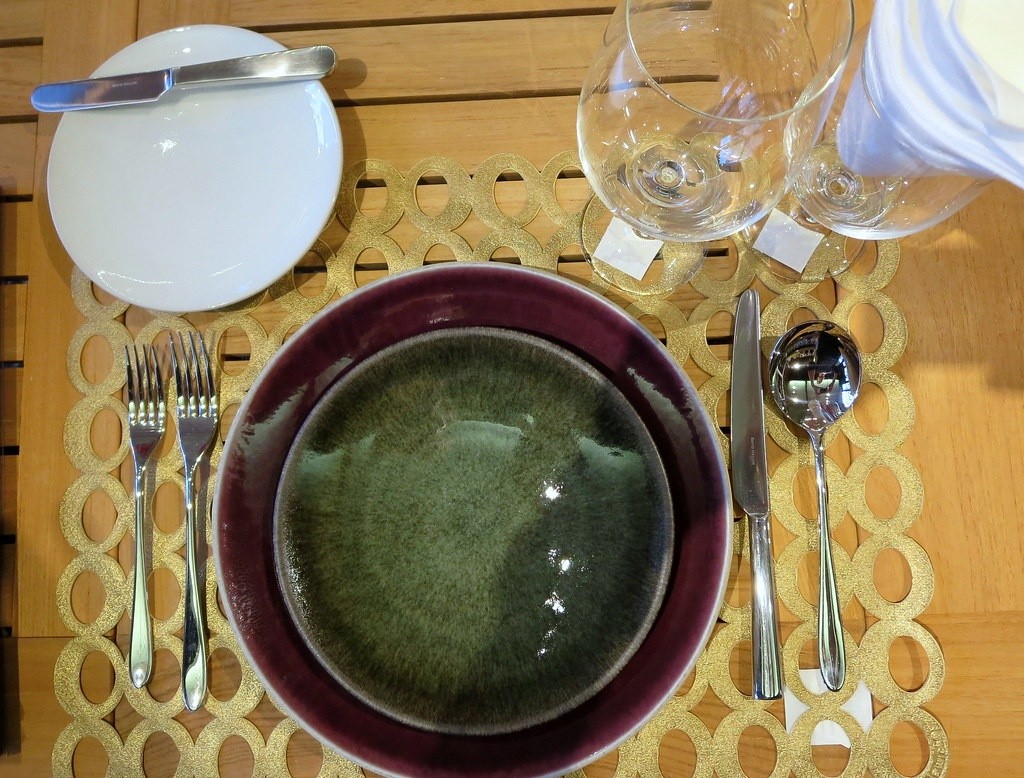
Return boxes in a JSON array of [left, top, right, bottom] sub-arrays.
[[125, 343, 164, 687], [167, 329, 220, 713]]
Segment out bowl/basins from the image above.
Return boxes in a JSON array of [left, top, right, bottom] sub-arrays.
[[211, 260, 733, 778]]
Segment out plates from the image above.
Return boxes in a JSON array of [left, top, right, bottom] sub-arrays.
[[45, 24, 342, 314]]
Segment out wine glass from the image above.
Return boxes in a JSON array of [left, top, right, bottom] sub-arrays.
[[738, 20, 997, 284], [575, 1, 855, 295]]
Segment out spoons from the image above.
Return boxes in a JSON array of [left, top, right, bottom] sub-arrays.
[[769, 320, 861, 691]]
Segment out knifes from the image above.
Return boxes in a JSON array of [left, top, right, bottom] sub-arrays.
[[31, 45, 341, 112], [730, 289, 782, 701]]
